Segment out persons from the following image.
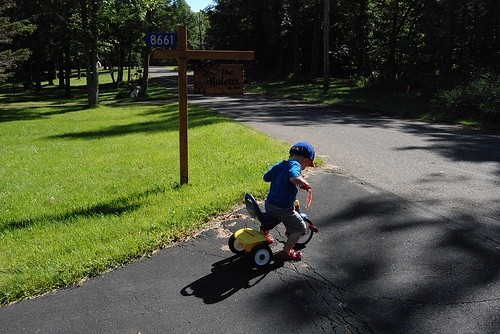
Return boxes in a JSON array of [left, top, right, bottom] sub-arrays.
[[260, 141, 316, 260]]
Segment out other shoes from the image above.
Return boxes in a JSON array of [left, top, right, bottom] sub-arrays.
[[263, 230, 276, 244], [280, 249, 302, 260]]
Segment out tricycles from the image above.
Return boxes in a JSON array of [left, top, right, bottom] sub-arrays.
[[229, 183, 318, 269]]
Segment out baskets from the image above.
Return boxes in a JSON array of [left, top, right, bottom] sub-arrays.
[[234, 227, 267, 252]]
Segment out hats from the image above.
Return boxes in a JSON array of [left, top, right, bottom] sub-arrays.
[[289, 142, 315, 168]]
